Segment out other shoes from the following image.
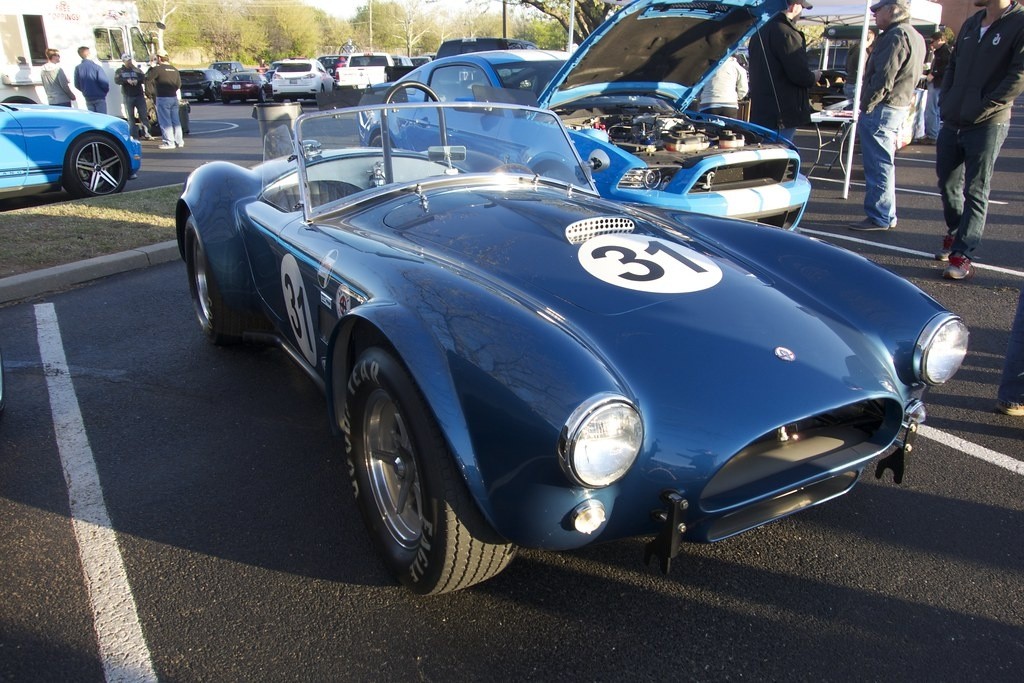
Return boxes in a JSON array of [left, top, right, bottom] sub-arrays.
[[889, 224, 896, 228], [178, 144, 184, 147], [918, 136, 937, 145], [848, 217, 889, 231], [158, 143, 176, 149], [146, 136, 155, 141]]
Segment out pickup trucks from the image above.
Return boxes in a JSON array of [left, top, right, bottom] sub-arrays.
[[337, 53, 395, 90]]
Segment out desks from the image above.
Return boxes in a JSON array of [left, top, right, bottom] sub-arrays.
[[804, 110, 860, 188]]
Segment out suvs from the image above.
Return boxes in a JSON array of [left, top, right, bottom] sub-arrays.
[[272, 56, 336, 105]]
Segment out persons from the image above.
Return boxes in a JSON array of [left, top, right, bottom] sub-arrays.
[[40, 48, 76, 107], [845, 0, 952, 231], [74, 47, 109, 115], [147, 48, 185, 150], [736, 0, 821, 150], [997, 287, 1024, 417], [935, 0, 1024, 280], [114, 53, 155, 141], [700, 55, 749, 118]]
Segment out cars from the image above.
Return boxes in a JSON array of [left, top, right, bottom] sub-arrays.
[[180, 62, 275, 105], [175, 100, 970, 598], [358, 0, 813, 231], [392, 55, 437, 66], [0, 102, 142, 206]]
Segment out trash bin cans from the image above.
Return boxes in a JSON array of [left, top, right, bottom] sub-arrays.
[[177, 102, 192, 134], [254, 102, 303, 157]]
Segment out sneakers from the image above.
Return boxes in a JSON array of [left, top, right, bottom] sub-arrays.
[[943, 253, 972, 279], [935, 234, 956, 261]]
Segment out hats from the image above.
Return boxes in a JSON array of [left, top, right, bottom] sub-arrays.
[[157, 49, 168, 58], [787, 0, 813, 10], [870, 0, 912, 13], [121, 54, 131, 61]]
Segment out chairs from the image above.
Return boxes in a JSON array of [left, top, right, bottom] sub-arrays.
[[270, 179, 364, 210]]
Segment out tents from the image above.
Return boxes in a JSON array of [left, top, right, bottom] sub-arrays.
[[788, 0, 946, 198]]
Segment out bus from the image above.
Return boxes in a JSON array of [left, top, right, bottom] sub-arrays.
[[0, 0, 166, 137]]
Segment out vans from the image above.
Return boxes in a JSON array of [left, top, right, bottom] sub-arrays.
[[806, 46, 850, 68], [435, 38, 538, 59]]
